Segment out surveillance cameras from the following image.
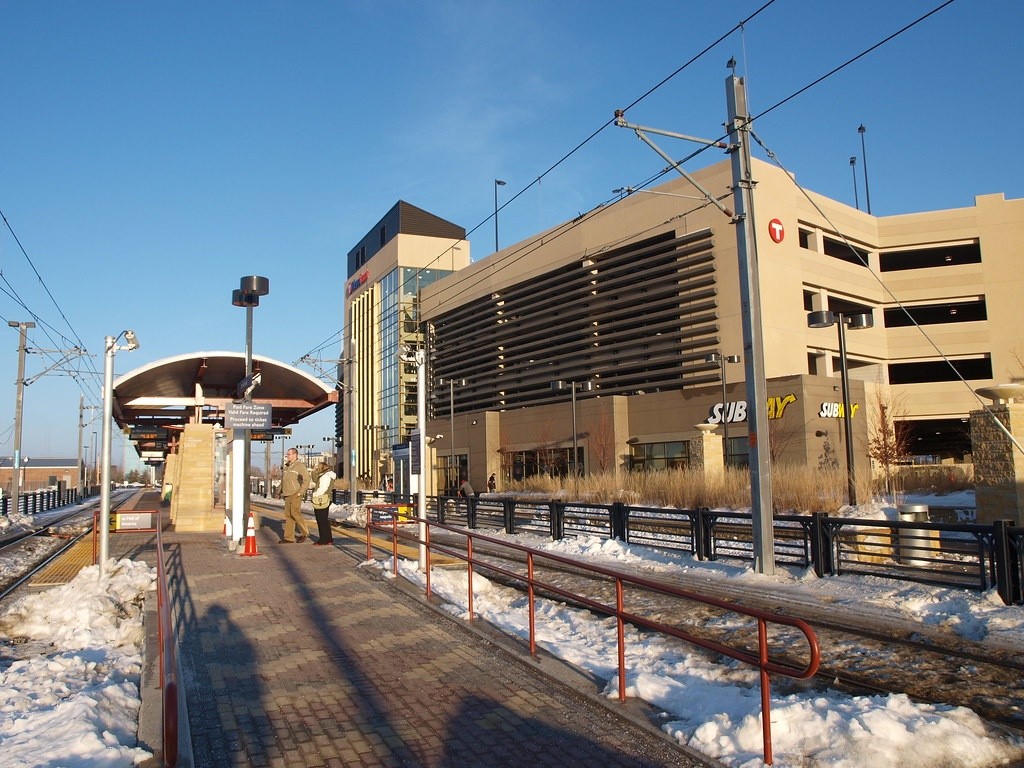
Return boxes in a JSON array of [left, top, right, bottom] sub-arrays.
[[125, 329, 140, 350]]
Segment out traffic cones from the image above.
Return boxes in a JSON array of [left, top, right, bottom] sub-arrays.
[[240, 512, 261, 556]]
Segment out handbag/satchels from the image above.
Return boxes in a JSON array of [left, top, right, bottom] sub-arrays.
[[311, 494, 329, 510]]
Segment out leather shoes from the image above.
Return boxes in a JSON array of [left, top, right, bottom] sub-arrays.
[[295, 534, 308, 543], [277, 538, 294, 544]]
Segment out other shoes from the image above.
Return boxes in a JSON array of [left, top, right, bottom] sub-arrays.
[[311, 540, 334, 546]]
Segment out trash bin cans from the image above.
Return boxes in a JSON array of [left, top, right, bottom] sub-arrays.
[[897, 503, 933, 567]]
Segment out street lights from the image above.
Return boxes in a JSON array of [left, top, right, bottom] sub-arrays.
[[276, 436, 291, 467], [261, 441, 274, 466], [551, 380, 597, 478], [706, 354, 742, 472], [231, 274, 270, 542], [435, 378, 468, 497], [296, 444, 314, 468], [364, 424, 390, 489], [323, 437, 342, 472], [807, 311, 875, 514]]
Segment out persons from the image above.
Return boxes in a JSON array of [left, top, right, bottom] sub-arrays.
[[487, 473, 497, 494], [274, 448, 311, 545], [311, 461, 337, 546], [458, 480, 475, 499]]
